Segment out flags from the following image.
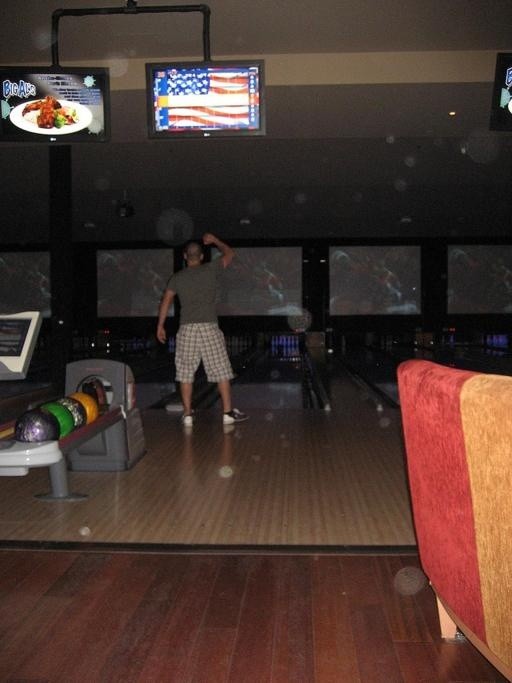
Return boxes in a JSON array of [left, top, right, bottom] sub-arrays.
[[150, 65, 261, 133]]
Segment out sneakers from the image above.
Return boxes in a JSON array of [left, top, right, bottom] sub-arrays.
[[179, 413, 195, 425], [222, 410, 251, 424]]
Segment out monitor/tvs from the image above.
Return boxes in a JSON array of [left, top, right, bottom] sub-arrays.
[[0, 310, 43, 380], [144, 59, 266, 139], [489, 52, 512, 132], [0, 65, 111, 143]]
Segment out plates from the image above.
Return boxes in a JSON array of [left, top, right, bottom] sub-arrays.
[[9, 98, 93, 136]]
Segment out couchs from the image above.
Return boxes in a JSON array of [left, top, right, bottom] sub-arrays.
[[394, 358, 510, 681]]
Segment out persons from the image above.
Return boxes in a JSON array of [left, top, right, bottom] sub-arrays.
[[157, 233, 250, 427]]
